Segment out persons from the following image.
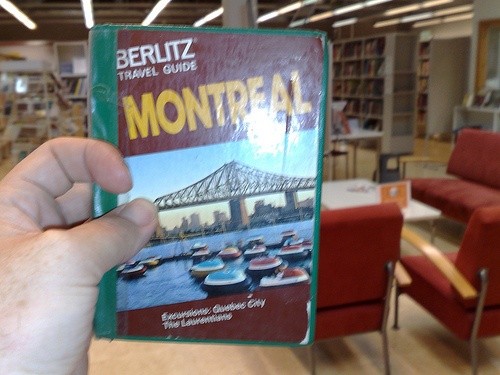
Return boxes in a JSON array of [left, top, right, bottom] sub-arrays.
[[0, 137, 157, 375]]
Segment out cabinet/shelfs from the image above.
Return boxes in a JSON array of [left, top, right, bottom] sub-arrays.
[[0, 41, 90, 169], [331, 29, 471, 150]]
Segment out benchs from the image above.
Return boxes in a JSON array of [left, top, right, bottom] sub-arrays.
[[406, 126, 500, 245]]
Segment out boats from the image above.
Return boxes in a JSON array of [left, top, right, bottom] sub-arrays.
[[247, 234, 266, 248], [115, 255, 161, 279], [202, 266, 253, 300], [258, 266, 309, 290], [188, 257, 225, 281], [275, 237, 313, 261], [190, 240, 210, 265], [244, 243, 270, 261], [217, 246, 242, 261], [245, 252, 289, 282], [279, 230, 297, 240]]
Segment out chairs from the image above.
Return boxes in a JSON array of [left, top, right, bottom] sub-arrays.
[[308, 201, 413, 375], [396, 202, 500, 375]]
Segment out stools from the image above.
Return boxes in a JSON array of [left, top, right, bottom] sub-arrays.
[[327, 149, 349, 180]]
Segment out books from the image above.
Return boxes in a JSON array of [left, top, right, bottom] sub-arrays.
[[416, 59, 428, 137], [86, 22, 328, 351], [62, 78, 87, 97], [332, 43, 341, 59], [332, 62, 342, 77], [363, 38, 383, 57], [343, 61, 361, 77], [0, 70, 82, 160], [419, 42, 430, 56], [363, 78, 384, 97], [343, 42, 362, 58], [363, 99, 383, 116], [363, 118, 384, 132], [332, 79, 343, 96], [462, 92, 500, 110], [347, 116, 361, 128], [343, 79, 361, 96], [343, 98, 360, 114], [364, 58, 385, 76]]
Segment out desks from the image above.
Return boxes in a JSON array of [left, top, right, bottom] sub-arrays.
[[450, 108, 500, 149], [331, 131, 384, 178], [321, 176, 442, 247]]
